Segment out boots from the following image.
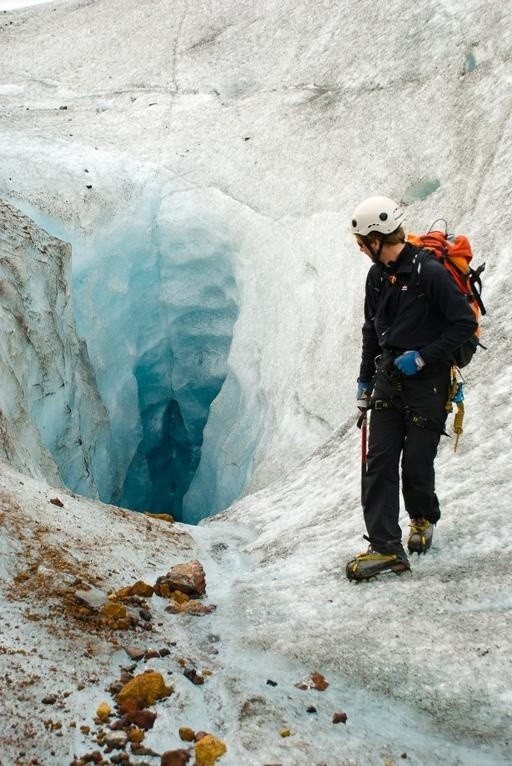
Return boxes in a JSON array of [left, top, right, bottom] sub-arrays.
[[407, 518, 434, 555], [346, 549, 409, 583]]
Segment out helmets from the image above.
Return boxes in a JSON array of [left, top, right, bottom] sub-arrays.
[[351, 197, 404, 235]]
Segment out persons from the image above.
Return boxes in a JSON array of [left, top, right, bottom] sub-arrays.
[[346, 195, 478, 580]]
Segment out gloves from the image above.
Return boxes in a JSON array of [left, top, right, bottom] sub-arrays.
[[355, 383, 371, 401], [393, 350, 425, 377]]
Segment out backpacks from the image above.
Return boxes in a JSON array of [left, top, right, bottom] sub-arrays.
[[415, 231, 484, 369]]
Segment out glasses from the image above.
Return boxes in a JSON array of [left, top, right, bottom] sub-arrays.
[[357, 240, 364, 248]]
